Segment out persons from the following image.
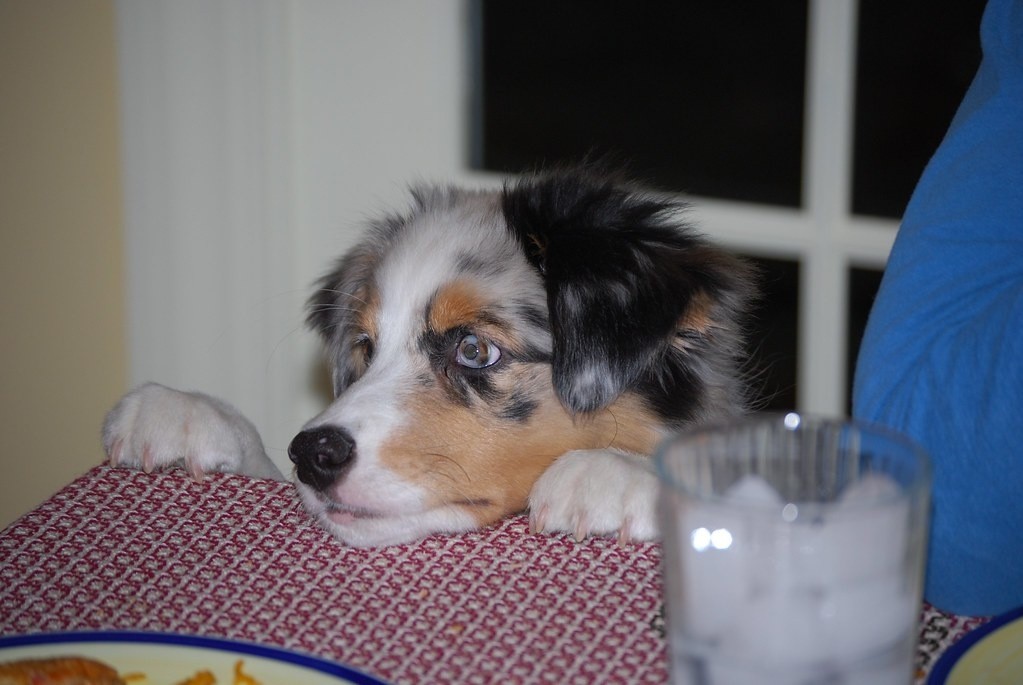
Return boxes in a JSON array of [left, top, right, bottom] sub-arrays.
[[851, 0, 1023, 619]]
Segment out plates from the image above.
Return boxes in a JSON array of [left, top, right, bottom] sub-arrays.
[[926, 606, 1023, 685], [0, 630, 393, 685]]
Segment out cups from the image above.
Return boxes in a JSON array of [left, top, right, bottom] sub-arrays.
[[652, 412, 931, 685]]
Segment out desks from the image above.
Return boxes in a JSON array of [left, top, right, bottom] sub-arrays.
[[0, 460, 1023, 685]]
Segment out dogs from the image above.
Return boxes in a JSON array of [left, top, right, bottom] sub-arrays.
[[99, 161, 760, 551]]
